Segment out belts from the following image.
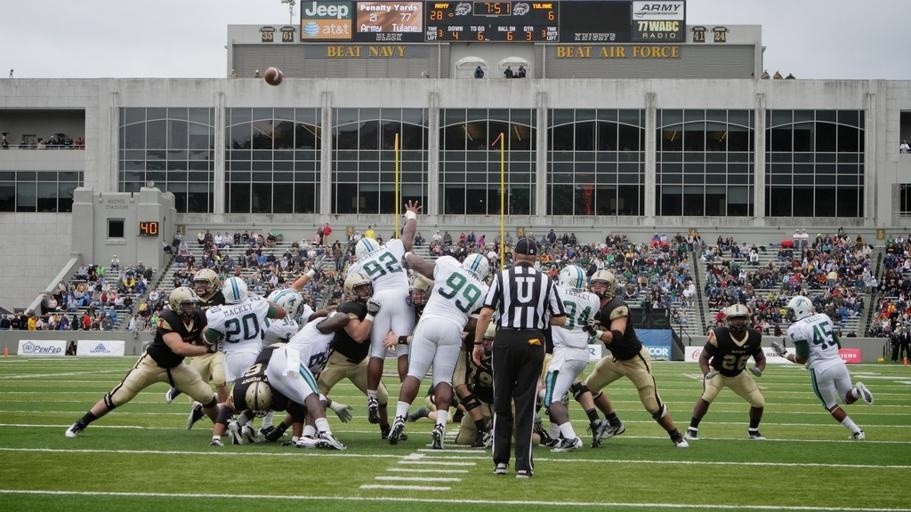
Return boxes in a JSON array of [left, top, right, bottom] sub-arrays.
[[495, 326, 544, 332]]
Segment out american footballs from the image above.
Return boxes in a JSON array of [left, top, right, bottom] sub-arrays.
[[262, 65, 285, 87]]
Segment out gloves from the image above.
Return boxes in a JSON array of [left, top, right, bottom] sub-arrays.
[[329, 398, 354, 425], [366, 297, 381, 313], [208, 340, 224, 354], [707, 372, 718, 380], [749, 366, 763, 378], [313, 254, 326, 273], [582, 324, 598, 339], [772, 340, 786, 358]]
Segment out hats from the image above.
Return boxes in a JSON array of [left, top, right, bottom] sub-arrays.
[[515, 239, 539, 257]]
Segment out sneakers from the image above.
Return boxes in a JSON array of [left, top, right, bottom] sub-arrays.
[[538, 435, 561, 449], [386, 417, 405, 444], [433, 422, 444, 450], [471, 431, 488, 447], [670, 435, 691, 450], [367, 398, 382, 425], [493, 461, 508, 476], [409, 409, 427, 423], [209, 437, 224, 450], [855, 380, 877, 407], [294, 435, 322, 450], [515, 469, 533, 480], [847, 430, 865, 445], [259, 426, 290, 442], [750, 433, 762, 438], [166, 386, 178, 403], [65, 421, 81, 440], [599, 421, 626, 439], [314, 432, 347, 451], [590, 420, 619, 448], [549, 438, 585, 456], [227, 422, 244, 446], [683, 430, 697, 443], [185, 399, 203, 431], [240, 425, 259, 445]]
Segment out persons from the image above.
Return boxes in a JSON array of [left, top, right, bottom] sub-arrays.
[[167, 268, 229, 403], [263, 274, 278, 297], [773, 297, 872, 441], [685, 305, 766, 439], [66, 286, 221, 438], [207, 275, 285, 383], [868, 235, 911, 364], [332, 224, 377, 272], [409, 383, 467, 425], [261, 257, 323, 345], [537, 229, 600, 278], [161, 228, 278, 278], [303, 274, 344, 310], [700, 226, 873, 307], [385, 274, 490, 448], [429, 231, 513, 271], [1, 255, 153, 331], [278, 223, 333, 277], [456, 322, 497, 446], [751, 304, 788, 336], [587, 270, 689, 448], [542, 266, 610, 453], [414, 231, 425, 247], [209, 341, 303, 448], [149, 290, 170, 331], [817, 305, 841, 322], [705, 310, 728, 334], [266, 311, 349, 450], [346, 199, 423, 425], [404, 251, 489, 449], [600, 229, 701, 307], [472, 239, 566, 479], [295, 272, 407, 448]]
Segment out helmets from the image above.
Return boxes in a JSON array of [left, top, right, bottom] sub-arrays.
[[405, 273, 435, 307], [192, 269, 224, 302], [169, 288, 206, 317], [221, 278, 250, 305], [784, 296, 816, 322], [266, 290, 284, 303], [557, 265, 588, 291], [589, 272, 618, 298], [462, 253, 490, 282], [273, 288, 304, 319], [343, 272, 377, 302], [724, 304, 753, 332], [485, 322, 496, 339], [245, 383, 275, 418], [327, 308, 338, 318], [355, 238, 380, 256]]
